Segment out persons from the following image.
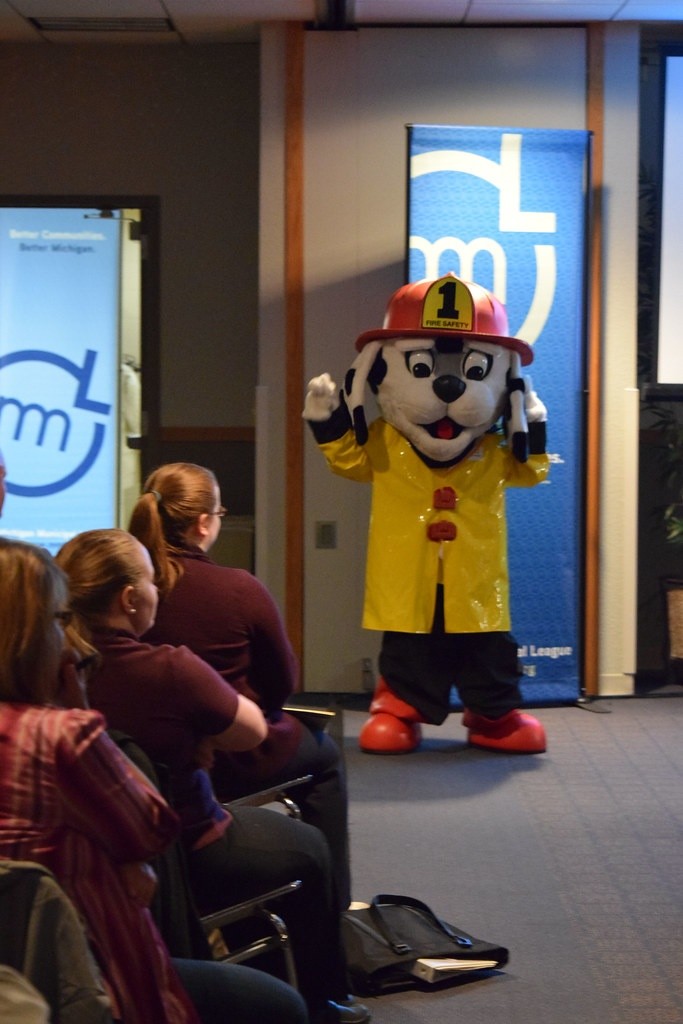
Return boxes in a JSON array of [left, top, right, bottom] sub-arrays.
[[128, 462, 379, 915], [0, 541, 311, 1023], [53, 530, 374, 1023]]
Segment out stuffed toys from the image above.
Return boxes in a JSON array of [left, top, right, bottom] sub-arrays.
[[301, 271, 547, 755]]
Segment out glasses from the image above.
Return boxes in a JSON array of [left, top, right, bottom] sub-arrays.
[[206, 506, 228, 517], [52, 610, 72, 628]]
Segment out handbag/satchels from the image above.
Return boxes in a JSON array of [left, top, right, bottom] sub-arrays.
[[339, 893, 510, 998]]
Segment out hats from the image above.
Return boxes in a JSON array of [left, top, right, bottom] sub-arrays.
[[354, 270, 534, 368]]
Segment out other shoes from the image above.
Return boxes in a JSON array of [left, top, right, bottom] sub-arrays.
[[328, 1000, 370, 1023], [462, 702, 547, 752], [357, 673, 426, 752]]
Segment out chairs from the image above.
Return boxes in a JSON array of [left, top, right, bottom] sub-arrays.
[[0, 767, 326, 1024]]
